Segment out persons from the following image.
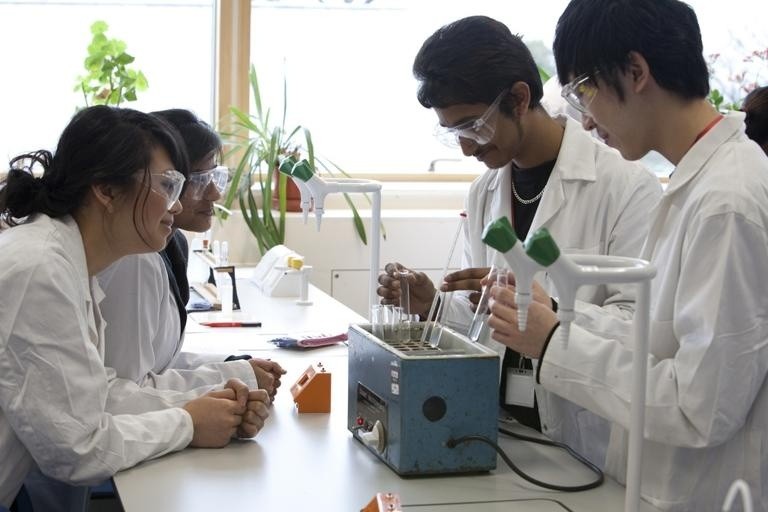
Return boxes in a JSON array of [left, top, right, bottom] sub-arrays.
[[376, 15, 663, 480], [739, 86, 768, 156], [469, 0, 768, 510], [9, 107, 288, 510], [0, 105, 270, 510]]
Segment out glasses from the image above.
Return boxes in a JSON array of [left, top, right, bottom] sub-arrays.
[[184, 163, 231, 203], [431, 84, 514, 148], [560, 49, 629, 116], [122, 168, 187, 205]]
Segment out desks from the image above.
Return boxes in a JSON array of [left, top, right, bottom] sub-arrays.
[[108, 268, 662, 512]]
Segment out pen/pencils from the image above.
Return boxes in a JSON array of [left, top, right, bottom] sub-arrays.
[[200, 322, 262, 327]]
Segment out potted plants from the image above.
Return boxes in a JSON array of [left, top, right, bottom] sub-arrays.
[[217, 63, 390, 261]]
